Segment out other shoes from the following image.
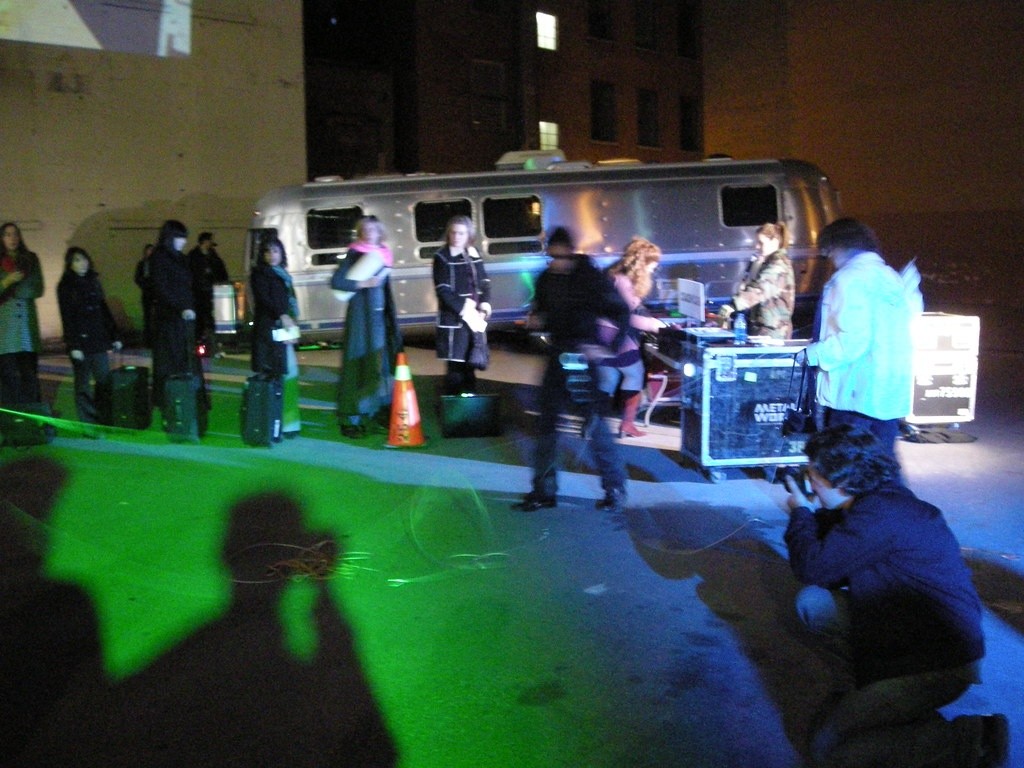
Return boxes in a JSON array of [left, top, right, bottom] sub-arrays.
[[597, 488, 628, 512], [982, 712, 1009, 768], [341, 424, 364, 438], [514, 492, 557, 513]]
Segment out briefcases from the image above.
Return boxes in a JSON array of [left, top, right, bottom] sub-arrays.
[[440, 393, 501, 439]]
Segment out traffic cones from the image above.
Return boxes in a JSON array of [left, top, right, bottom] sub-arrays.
[[385, 353, 429, 451]]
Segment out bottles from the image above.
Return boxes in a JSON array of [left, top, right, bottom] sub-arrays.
[[733, 313, 747, 346]]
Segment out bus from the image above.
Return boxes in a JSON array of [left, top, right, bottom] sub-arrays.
[[241, 149, 850, 344]]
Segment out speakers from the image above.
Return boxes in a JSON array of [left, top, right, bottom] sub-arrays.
[[440, 391, 502, 437], [103, 366, 151, 430]]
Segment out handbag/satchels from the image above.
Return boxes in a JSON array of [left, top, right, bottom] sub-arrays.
[[0, 402, 56, 446]]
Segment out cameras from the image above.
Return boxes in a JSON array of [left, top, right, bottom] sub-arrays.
[[784, 469, 815, 496]]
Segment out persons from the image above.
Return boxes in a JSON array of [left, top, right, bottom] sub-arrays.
[[512, 226, 630, 511], [586, 235, 667, 439], [0, 222, 46, 401], [251, 240, 302, 440], [332, 215, 405, 438], [134, 220, 230, 429], [785, 423, 1009, 768], [718, 219, 797, 339], [793, 218, 915, 449], [433, 217, 491, 394], [56, 247, 122, 426]]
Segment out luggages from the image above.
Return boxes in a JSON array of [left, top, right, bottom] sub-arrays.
[[108, 342, 150, 428], [163, 315, 198, 443], [240, 371, 286, 447]]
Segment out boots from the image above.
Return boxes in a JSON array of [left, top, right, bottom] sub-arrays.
[[618, 389, 647, 438]]
